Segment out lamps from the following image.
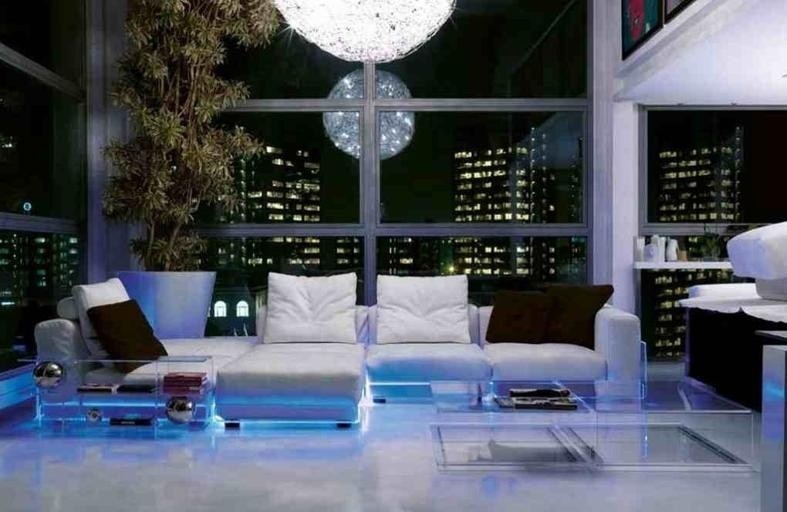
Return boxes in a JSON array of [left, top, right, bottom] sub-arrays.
[[270, 0, 463, 63], [318, 68, 415, 162]]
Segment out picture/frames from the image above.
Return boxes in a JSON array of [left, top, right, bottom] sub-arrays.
[[620, 0, 664, 62], [663, 0, 696, 25]]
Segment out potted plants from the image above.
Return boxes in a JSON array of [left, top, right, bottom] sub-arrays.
[[104, 1, 276, 345]]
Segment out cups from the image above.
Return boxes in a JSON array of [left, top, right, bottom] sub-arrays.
[[677, 250, 687, 261]]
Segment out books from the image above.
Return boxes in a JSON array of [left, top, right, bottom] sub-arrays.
[[110, 413, 155, 425], [164, 372, 210, 395], [77, 383, 120, 393], [117, 383, 160, 393], [508, 388, 577, 409]]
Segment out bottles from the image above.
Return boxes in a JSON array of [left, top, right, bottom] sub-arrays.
[[664, 239, 678, 261]]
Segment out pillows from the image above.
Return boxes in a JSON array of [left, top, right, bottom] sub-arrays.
[[262, 270, 361, 345], [479, 285, 558, 347], [534, 278, 616, 354], [374, 272, 470, 345], [71, 278, 141, 365], [111, 268, 221, 342], [83, 299, 168, 376]]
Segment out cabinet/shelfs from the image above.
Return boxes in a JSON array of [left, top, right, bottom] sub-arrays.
[[630, 257, 757, 363], [679, 277, 787, 415]]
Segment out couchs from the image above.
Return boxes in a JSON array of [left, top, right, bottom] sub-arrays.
[[33, 268, 649, 432]]
